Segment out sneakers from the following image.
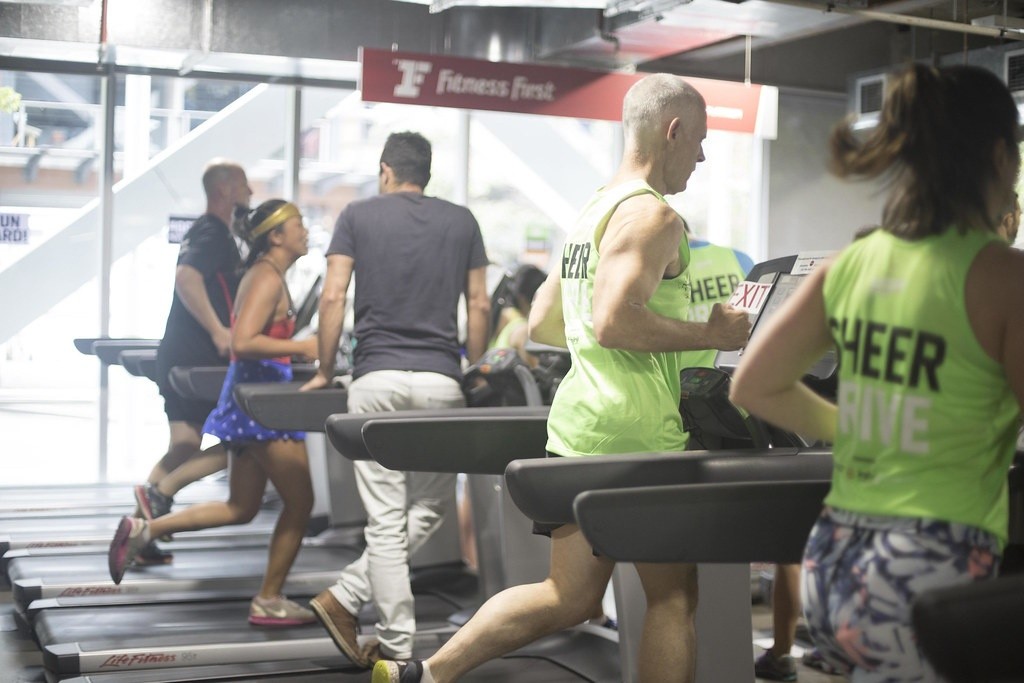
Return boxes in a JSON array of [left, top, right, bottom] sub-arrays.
[[134, 483, 174, 542], [361, 640, 392, 668], [248, 594, 317, 625], [755, 648, 797, 683], [309, 589, 370, 670], [371, 660, 423, 683], [802, 649, 844, 674], [109, 516, 150, 586]]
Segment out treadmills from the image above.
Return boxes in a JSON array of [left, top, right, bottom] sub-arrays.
[[0, 253, 1024, 683]]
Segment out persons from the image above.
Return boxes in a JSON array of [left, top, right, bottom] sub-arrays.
[[128, 164, 253, 568], [727, 63, 1024, 682], [679, 213, 756, 372], [368, 73, 749, 683], [309, 130, 490, 670], [754, 562, 846, 682], [458, 264, 549, 571], [108, 200, 317, 625]]
